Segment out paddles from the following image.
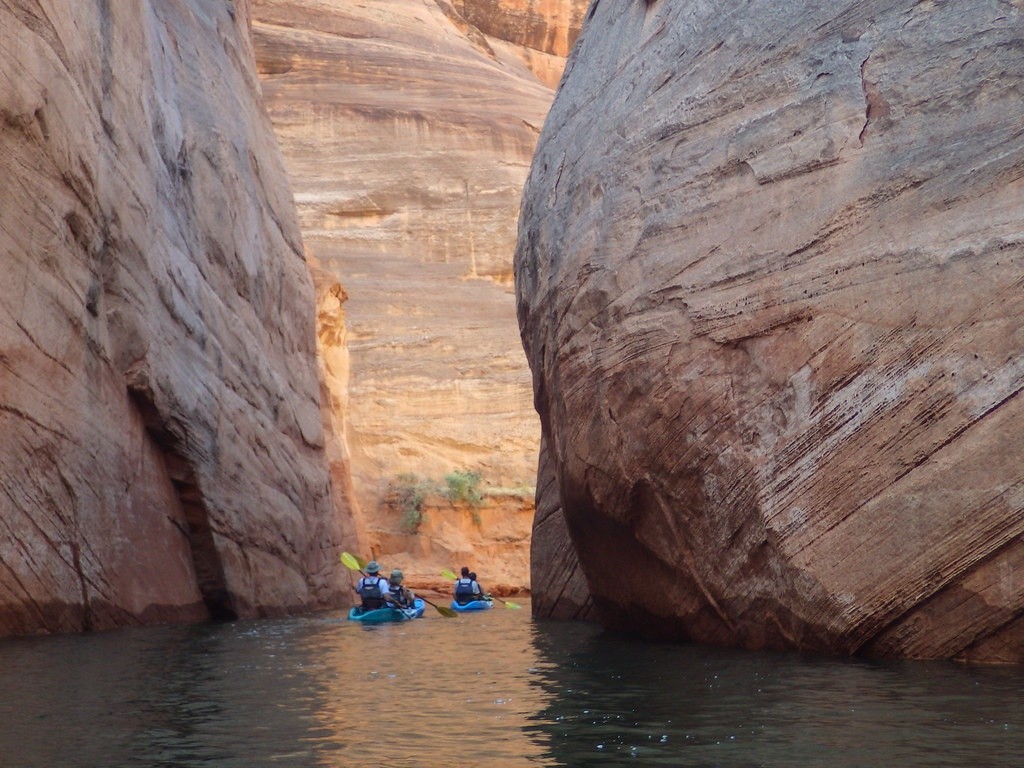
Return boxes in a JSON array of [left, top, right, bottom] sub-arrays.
[[340, 552, 405, 608], [379, 573, 457, 617], [439, 569, 520, 608]]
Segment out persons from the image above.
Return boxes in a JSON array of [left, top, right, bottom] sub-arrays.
[[469, 572, 484, 594], [453, 567, 482, 601], [351, 561, 407, 610], [386, 571, 416, 609]]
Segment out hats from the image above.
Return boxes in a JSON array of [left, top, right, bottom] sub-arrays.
[[389, 570, 402, 583], [364, 561, 381, 573]]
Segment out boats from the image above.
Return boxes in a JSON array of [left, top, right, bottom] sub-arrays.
[[448, 597, 492, 613], [349, 599, 425, 624]]
